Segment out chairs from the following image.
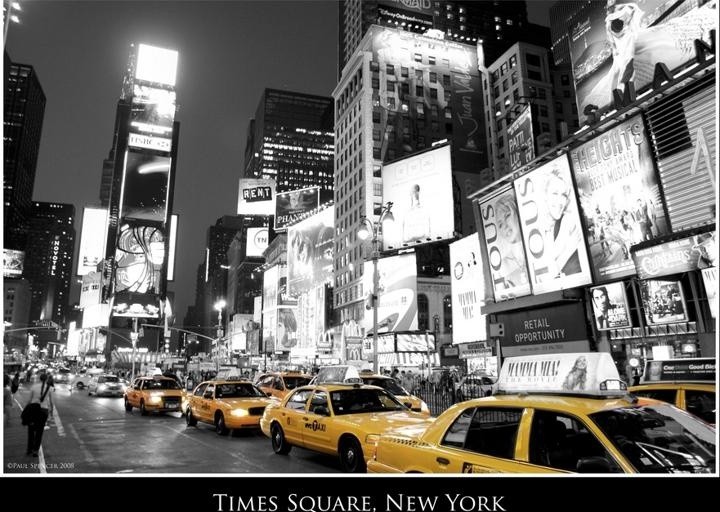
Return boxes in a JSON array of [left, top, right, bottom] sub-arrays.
[[475, 419, 616, 471]]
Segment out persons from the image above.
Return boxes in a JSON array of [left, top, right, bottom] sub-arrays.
[[277, 308, 297, 347], [4, 375, 12, 422], [496, 194, 522, 245], [646, 285, 678, 323], [27, 373, 54, 457], [591, 287, 618, 329], [289, 230, 334, 273], [594, 199, 659, 261], [605, 13, 633, 100], [10, 373, 20, 400], [692, 233, 716, 268], [601, 0, 634, 43], [385, 368, 439, 394], [539, 166, 582, 278], [561, 355, 588, 391]]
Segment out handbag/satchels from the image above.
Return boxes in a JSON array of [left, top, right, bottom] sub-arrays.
[[21, 403, 40, 425]]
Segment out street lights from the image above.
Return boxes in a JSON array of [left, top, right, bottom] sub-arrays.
[[212, 294, 226, 374], [353, 200, 397, 375]]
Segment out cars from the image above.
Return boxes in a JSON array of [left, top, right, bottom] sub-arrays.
[[366, 349, 716, 477], [449, 373, 496, 400], [614, 357, 719, 433], [21, 360, 437, 474]]
